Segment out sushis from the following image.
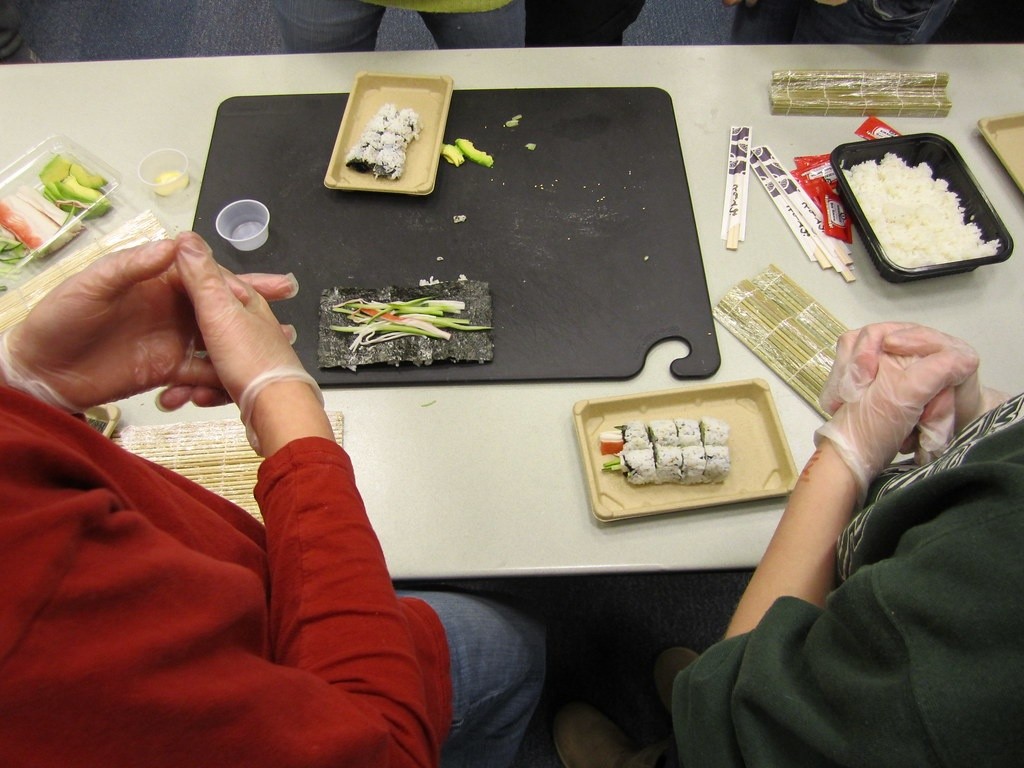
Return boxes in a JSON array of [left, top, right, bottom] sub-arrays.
[[342, 103, 422, 180], [597, 417, 732, 484]]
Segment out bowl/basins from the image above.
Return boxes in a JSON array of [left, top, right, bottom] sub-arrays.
[[137, 148, 189, 196], [215, 200, 271, 254]]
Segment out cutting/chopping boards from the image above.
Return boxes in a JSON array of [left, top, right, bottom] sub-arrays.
[[191, 86, 721, 385]]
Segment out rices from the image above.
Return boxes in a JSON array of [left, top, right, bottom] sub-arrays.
[[841, 151, 1003, 269]]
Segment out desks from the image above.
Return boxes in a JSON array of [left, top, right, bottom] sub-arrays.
[[0, 42, 1024, 580]]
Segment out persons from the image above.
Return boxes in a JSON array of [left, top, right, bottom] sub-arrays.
[[0, 233, 549, 768], [550, 321, 1024, 768], [272, 0, 526, 53], [721, 0, 958, 45]]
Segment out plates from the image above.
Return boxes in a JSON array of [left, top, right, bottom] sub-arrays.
[[976, 112, 1024, 192], [322, 71, 454, 194], [574, 379, 798, 521], [830, 133, 1014, 282]]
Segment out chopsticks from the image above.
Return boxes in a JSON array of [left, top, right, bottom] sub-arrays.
[[720, 126, 855, 285]]
